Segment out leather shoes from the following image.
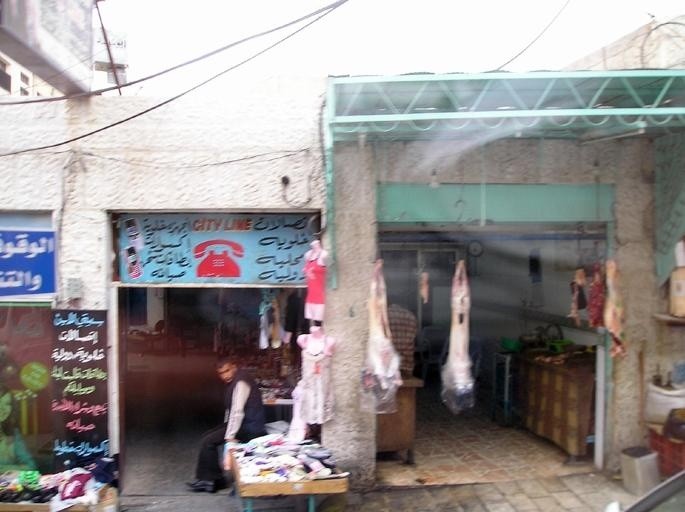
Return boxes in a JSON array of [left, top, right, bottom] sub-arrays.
[[185, 481, 215, 492]]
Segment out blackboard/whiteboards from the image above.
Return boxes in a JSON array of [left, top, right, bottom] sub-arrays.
[[49, 308, 108, 475]]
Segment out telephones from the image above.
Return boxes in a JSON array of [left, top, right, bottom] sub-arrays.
[[194, 239, 244, 277]]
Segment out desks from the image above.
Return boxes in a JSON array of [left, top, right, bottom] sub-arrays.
[[375, 377, 426, 464]]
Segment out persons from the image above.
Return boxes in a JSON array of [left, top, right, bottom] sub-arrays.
[[186, 360, 267, 492]]
